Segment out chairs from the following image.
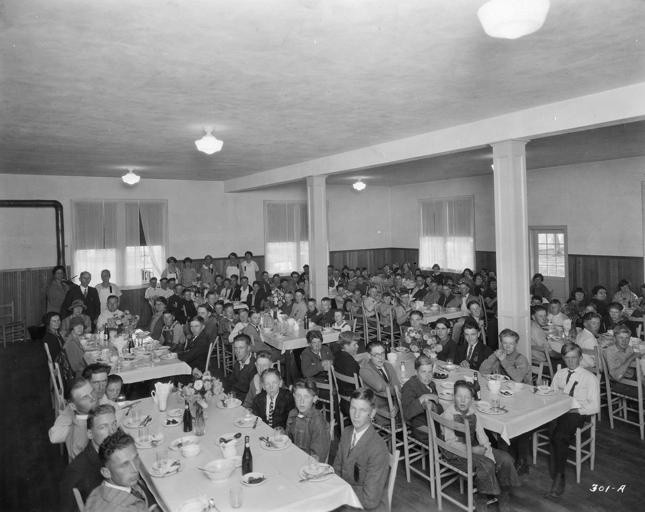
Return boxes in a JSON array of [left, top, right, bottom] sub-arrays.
[[427, 407, 498, 512], [361, 302, 387, 345], [0, 299, 27, 349], [481, 296, 495, 330], [358, 375, 426, 478], [599, 348, 645, 440], [330, 365, 359, 435], [375, 306, 402, 348], [533, 363, 602, 484], [217, 321, 235, 377], [48, 361, 66, 456], [351, 301, 376, 346], [581, 346, 622, 429], [43, 342, 57, 410], [531, 346, 555, 382]]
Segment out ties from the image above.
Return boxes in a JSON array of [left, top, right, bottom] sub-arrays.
[[268, 396, 275, 428], [83, 288, 87, 297], [566, 370, 575, 384], [130, 486, 143, 500], [379, 368, 389, 384], [466, 345, 473, 360]]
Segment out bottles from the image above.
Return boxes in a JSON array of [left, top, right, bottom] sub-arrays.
[[129, 333, 135, 355], [474, 372, 481, 401], [242, 436, 253, 476], [183, 400, 192, 432], [103, 323, 109, 340], [304, 313, 308, 329], [401, 361, 405, 371]]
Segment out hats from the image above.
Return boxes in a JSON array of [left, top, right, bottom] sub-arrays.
[[68, 298, 87, 312], [68, 316, 85, 329]]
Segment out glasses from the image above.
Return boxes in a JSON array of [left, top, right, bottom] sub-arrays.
[[369, 351, 386, 358]]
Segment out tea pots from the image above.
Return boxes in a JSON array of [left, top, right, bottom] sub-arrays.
[[151, 385, 170, 412]]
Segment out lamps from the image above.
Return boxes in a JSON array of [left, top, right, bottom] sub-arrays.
[[352, 179, 366, 191], [476, 0, 550, 39], [121, 169, 141, 185], [194, 126, 224, 155]]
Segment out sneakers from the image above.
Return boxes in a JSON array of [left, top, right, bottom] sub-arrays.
[[497, 489, 512, 512], [548, 474, 567, 498], [473, 495, 499, 512], [515, 463, 530, 475]]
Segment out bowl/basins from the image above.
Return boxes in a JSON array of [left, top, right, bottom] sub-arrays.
[[538, 385, 553, 393], [241, 472, 271, 487], [216, 431, 240, 457], [182, 444, 202, 458], [499, 390, 514, 397], [204, 459, 234, 482], [163, 418, 182, 427], [272, 436, 289, 448]]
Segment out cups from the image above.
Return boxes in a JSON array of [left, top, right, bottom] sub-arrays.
[[117, 395, 126, 406], [543, 379, 548, 388], [492, 399, 500, 413], [308, 455, 319, 473], [274, 426, 284, 442], [139, 426, 149, 442], [130, 408, 142, 423], [229, 486, 243, 509], [488, 381, 499, 394], [447, 358, 454, 366], [245, 409, 253, 422], [228, 391, 235, 404], [155, 449, 169, 468]]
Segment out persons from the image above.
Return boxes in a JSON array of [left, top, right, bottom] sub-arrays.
[[222, 335, 256, 402], [453, 320, 493, 371], [145, 277, 159, 307], [517, 342, 600, 498], [214, 300, 224, 320], [179, 289, 197, 316], [84, 427, 157, 511], [335, 331, 362, 388], [327, 261, 498, 324], [161, 256, 180, 284], [58, 316, 86, 377], [170, 284, 183, 306], [167, 278, 176, 297], [401, 354, 444, 445], [242, 351, 272, 411], [438, 380, 522, 512], [212, 275, 222, 293], [182, 257, 196, 288], [530, 274, 603, 310], [63, 271, 101, 334], [399, 310, 432, 347], [219, 251, 330, 350], [174, 315, 210, 387], [252, 368, 294, 429], [157, 310, 186, 351], [574, 312, 602, 368], [199, 292, 216, 312], [458, 301, 487, 346], [196, 255, 217, 284], [603, 280, 645, 335], [95, 270, 122, 312], [159, 278, 168, 297], [49, 363, 118, 444], [58, 404, 118, 512], [530, 305, 562, 375], [64, 377, 98, 464], [41, 311, 60, 356], [301, 330, 334, 384], [147, 296, 168, 340], [363, 341, 402, 424], [433, 317, 455, 362], [97, 296, 123, 331], [604, 324, 641, 398], [105, 374, 123, 400], [196, 304, 217, 341], [46, 266, 70, 313], [62, 299, 91, 334], [332, 388, 389, 512], [285, 377, 331, 463], [480, 328, 532, 385]]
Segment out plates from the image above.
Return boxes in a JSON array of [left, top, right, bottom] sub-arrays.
[[300, 463, 335, 482], [152, 459, 180, 477], [168, 408, 186, 417], [123, 415, 151, 428], [170, 435, 201, 451], [485, 407, 507, 415], [438, 390, 456, 402], [217, 396, 242, 409], [235, 415, 261, 429], [133, 435, 163, 449], [441, 382, 457, 389], [260, 435, 292, 451]]
